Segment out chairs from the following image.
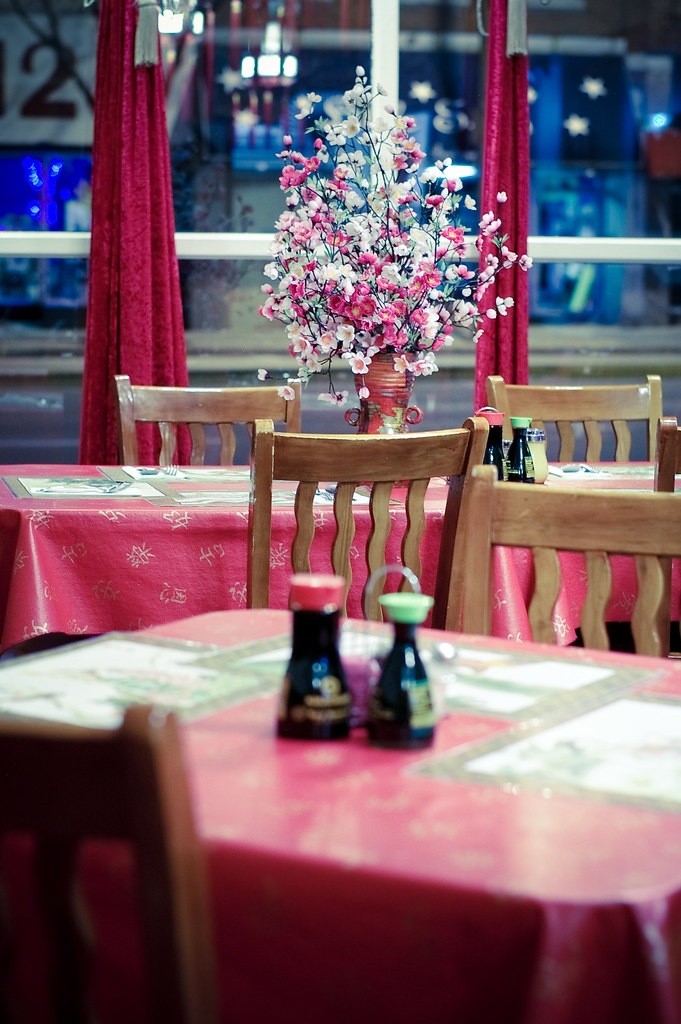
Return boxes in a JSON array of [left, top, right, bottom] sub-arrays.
[[0, 703, 220, 1024], [114, 374, 301, 467], [486, 374, 662, 462], [246, 417, 489, 631], [653, 416, 681, 658], [446, 464, 681, 658]]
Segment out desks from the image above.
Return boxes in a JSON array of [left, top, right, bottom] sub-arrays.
[[0, 462, 681, 648], [0, 607, 681, 1024]]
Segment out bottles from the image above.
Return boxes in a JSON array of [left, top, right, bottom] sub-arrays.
[[474, 406, 505, 481], [365, 593, 435, 750], [526, 428, 549, 484], [274, 571, 354, 744], [505, 417, 536, 484]]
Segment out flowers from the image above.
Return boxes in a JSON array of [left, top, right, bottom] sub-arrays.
[[256, 66, 535, 407]]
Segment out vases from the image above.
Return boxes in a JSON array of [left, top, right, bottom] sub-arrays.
[[344, 353, 423, 488]]
